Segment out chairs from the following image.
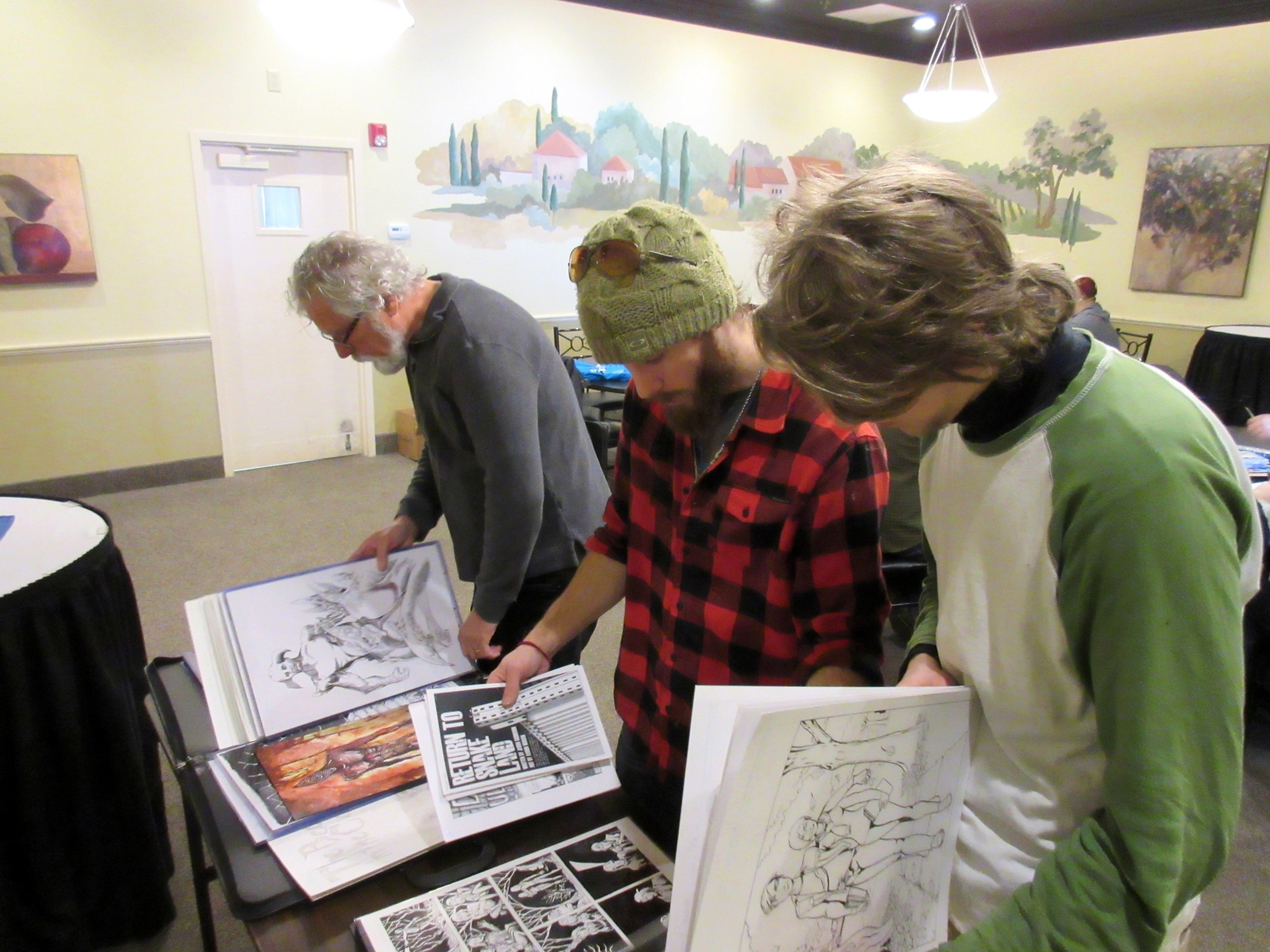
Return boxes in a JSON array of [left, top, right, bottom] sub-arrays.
[[554, 326, 622, 466], [1117, 326, 1154, 364]]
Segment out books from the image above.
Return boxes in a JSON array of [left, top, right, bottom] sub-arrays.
[[347, 816, 674, 952], [184, 544, 621, 902], [1236, 444, 1270, 476]]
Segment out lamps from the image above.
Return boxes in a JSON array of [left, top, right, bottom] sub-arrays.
[[903, 1, 998, 123]]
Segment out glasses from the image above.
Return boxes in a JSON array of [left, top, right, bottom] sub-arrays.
[[567, 238, 697, 283], [321, 309, 365, 347]]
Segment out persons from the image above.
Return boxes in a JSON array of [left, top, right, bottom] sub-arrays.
[[1254, 481, 1270, 503], [1048, 263, 1064, 272], [1247, 413, 1270, 441], [751, 156, 1264, 952], [284, 234, 611, 675], [871, 421, 919, 620], [1067, 276, 1119, 349], [486, 197, 888, 849]]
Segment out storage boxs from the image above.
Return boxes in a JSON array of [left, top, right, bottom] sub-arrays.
[[397, 408, 426, 462]]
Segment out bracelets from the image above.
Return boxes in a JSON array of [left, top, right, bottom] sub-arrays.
[[899, 643, 939, 677], [517, 641, 551, 665]]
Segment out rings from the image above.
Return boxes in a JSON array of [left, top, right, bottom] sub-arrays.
[[474, 650, 480, 652]]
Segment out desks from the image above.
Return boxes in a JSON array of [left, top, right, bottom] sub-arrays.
[[1186, 325, 1270, 426], [143, 690, 675, 952], [583, 379, 630, 393], [0, 490, 178, 952]]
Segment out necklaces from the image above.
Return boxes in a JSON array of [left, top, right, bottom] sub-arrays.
[[694, 359, 763, 479]]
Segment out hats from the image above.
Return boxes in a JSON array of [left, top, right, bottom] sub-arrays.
[[576, 200, 737, 365], [1076, 277, 1097, 298]]
